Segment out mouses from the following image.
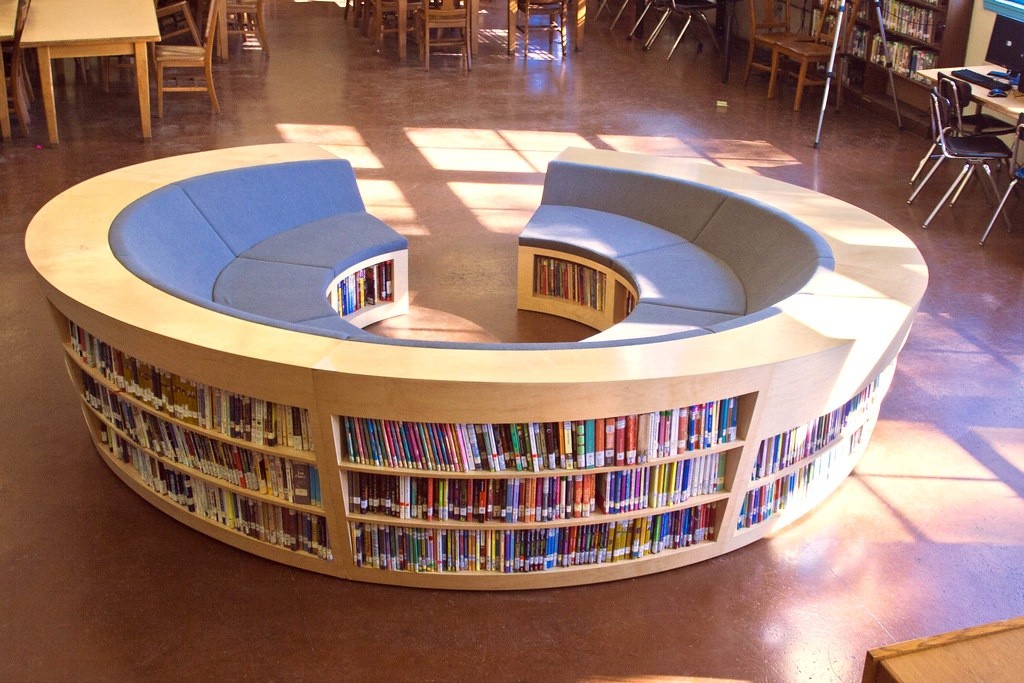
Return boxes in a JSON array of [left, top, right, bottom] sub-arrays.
[[988, 88, 1008, 97]]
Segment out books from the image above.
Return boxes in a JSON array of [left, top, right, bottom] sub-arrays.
[[337, 260, 392, 317], [636, 397, 739, 559], [811, 0, 867, 58], [499, 416, 644, 573], [117, 421, 333, 562], [869, 33, 938, 86], [342, 416, 506, 572], [69, 318, 317, 452], [536, 257, 607, 312], [882, 0, 946, 46], [624, 291, 635, 317], [736, 377, 880, 532]]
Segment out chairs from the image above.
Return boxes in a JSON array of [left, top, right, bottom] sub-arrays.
[[907, 72, 1024, 246], [342, 0, 473, 71], [594, 0, 723, 60], [742, 0, 861, 112], [515, 2, 573, 60], [3, 1, 271, 136]]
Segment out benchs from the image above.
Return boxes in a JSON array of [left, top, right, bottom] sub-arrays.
[[109, 157, 835, 351]]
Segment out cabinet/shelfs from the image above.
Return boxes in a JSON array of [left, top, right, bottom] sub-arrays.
[[810, 1, 975, 138], [736, 351, 897, 534], [330, 392, 764, 577], [45, 297, 333, 576]]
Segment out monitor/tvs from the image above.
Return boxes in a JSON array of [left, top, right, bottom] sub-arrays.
[[985, 13, 1024, 85]]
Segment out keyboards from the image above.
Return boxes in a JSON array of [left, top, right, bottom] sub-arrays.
[[951, 69, 1011, 91]]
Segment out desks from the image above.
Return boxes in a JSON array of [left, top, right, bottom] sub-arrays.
[[917, 64, 1024, 121], [0, 0, 18, 138], [19, 0, 162, 145]]
